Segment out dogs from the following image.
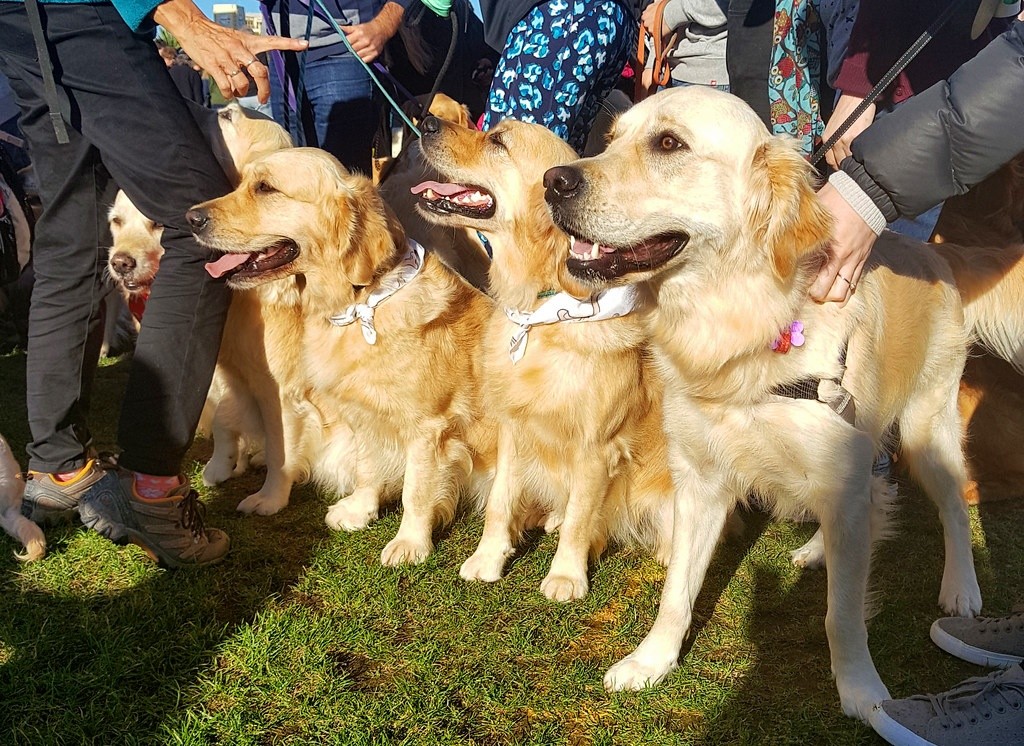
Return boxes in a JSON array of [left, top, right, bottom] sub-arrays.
[[0, 86, 1024, 726]]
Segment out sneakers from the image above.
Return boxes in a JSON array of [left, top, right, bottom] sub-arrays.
[[77, 466, 231, 571], [23, 459, 110, 512], [868, 610, 1024, 745]]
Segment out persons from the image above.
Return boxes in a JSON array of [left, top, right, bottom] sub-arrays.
[[0, 72, 44, 355], [809, 0, 1024, 746], [257, 0, 1023, 480], [235, 27, 272, 120], [0, 0, 311, 571], [156, 39, 212, 108]]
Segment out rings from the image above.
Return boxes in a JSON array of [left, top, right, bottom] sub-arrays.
[[231, 70, 241, 77], [838, 274, 855, 290], [247, 60, 259, 69]]
